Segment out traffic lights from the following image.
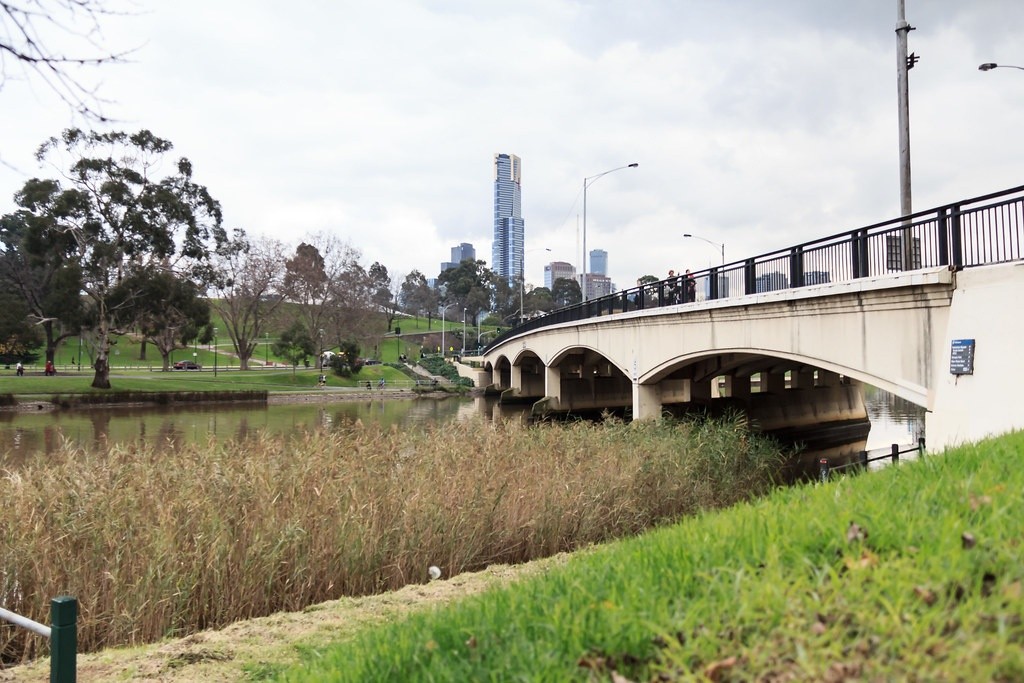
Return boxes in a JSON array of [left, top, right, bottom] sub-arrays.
[[497, 327, 499, 333]]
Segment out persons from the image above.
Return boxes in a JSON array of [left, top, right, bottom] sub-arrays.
[[379, 378, 384, 389], [432, 378, 438, 391], [666, 270, 681, 305], [399, 353, 406, 363], [71, 354, 76, 365], [45, 360, 54, 376], [366, 380, 371, 390], [317, 373, 327, 386], [16, 361, 24, 376], [684, 269, 697, 303]]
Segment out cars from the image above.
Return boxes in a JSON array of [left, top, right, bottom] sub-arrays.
[[174, 360, 202, 369]]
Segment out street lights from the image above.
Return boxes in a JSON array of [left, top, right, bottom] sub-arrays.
[[583, 163, 638, 303], [265, 333, 268, 365], [170, 327, 174, 371], [520, 248, 551, 324], [442, 302, 458, 357], [685, 234, 725, 297], [464, 308, 468, 350], [319, 329, 324, 372], [213, 328, 218, 377]]
